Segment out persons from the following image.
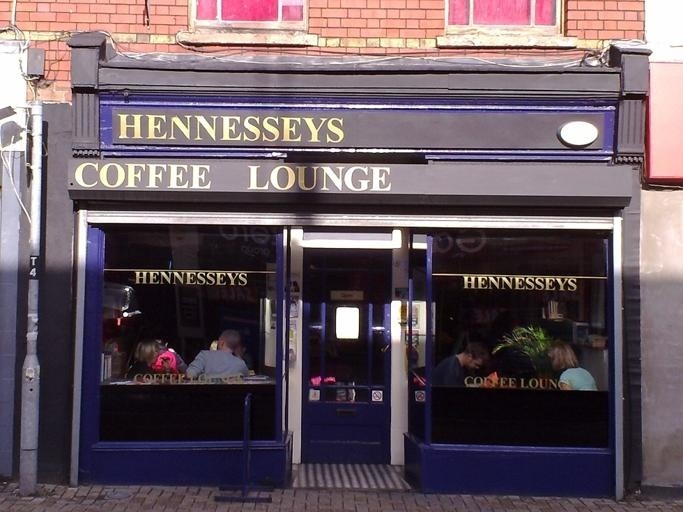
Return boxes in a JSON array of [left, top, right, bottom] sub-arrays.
[[547, 338, 600, 391], [125, 339, 169, 381], [235, 343, 253, 369], [184, 329, 249, 379], [431, 339, 490, 388]]
